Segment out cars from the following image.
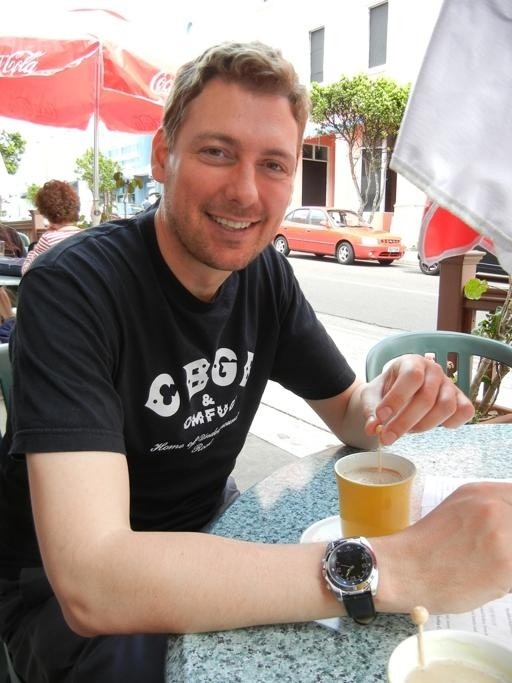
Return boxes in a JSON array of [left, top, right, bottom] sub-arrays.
[[271, 206, 406, 266], [418, 244, 511, 282]]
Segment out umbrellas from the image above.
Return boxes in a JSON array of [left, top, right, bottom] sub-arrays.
[[0, 0, 179, 228]]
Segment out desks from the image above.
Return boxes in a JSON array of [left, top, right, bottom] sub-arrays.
[[162, 420, 512, 680]]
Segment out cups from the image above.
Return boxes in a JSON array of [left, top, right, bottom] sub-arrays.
[[386, 629, 512, 683], [334, 451, 417, 538]]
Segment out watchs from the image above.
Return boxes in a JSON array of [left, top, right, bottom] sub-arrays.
[[321, 534, 379, 625]]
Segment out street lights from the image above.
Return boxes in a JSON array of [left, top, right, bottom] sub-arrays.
[[351, 145, 388, 213]]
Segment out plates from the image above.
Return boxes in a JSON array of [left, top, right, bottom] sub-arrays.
[[299, 513, 343, 544]]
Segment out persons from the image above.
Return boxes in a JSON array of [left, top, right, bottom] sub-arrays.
[[0, 35, 509, 682], [0, 177, 88, 345], [0, 222, 27, 257]]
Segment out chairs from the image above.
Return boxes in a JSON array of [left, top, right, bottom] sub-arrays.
[[362, 329, 511, 426]]
